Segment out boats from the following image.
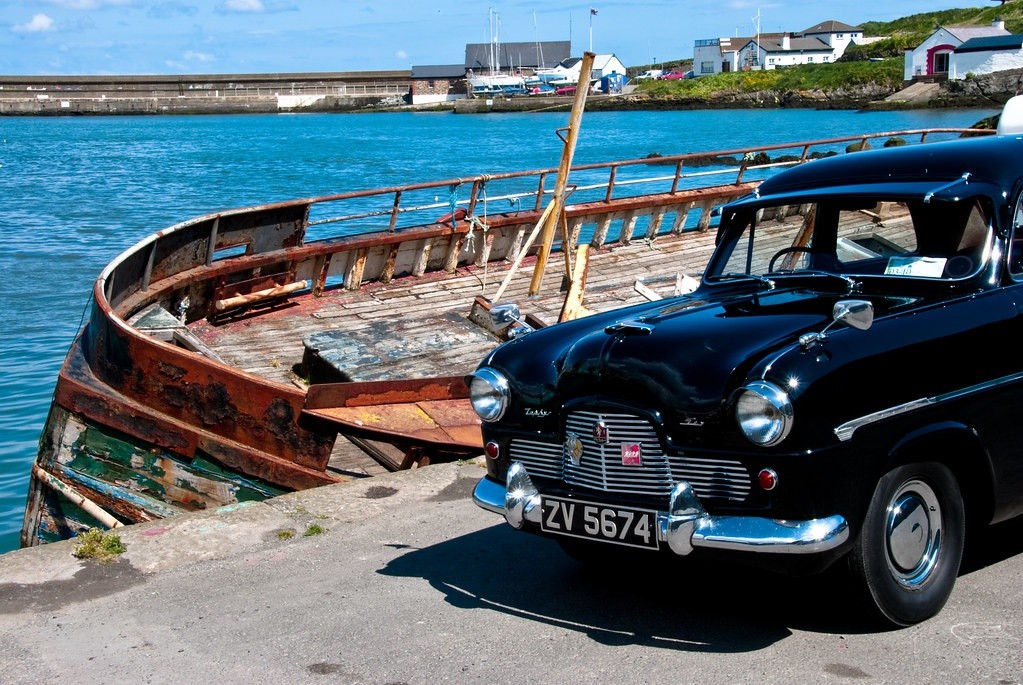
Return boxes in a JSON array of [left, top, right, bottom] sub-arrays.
[[20, 128, 998, 549]]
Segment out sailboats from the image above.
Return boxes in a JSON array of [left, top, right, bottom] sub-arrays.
[[461, 7, 528, 99]]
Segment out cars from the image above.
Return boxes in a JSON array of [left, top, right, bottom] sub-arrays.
[[684, 70, 694, 79], [462, 133, 1023, 627], [528, 85, 555, 97], [656, 69, 684, 80], [556, 85, 595, 95]]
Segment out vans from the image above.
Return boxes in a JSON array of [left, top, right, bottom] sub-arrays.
[[635, 70, 662, 81]]
